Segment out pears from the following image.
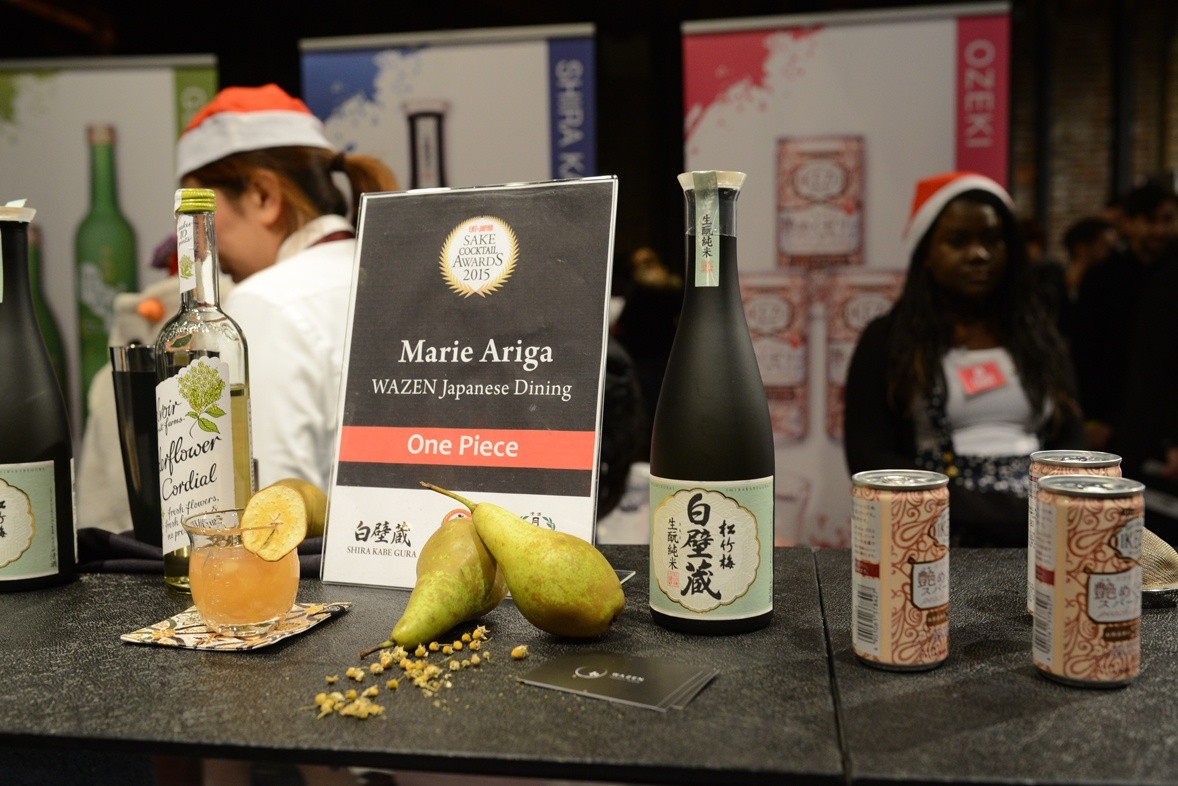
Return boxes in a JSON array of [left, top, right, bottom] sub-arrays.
[[417, 478, 627, 640], [360, 516, 506, 659]]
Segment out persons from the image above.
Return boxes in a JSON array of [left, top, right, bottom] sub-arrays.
[[177, 84, 406, 529], [609, 239, 682, 401], [835, 166, 1088, 545], [1014, 175, 1178, 496]]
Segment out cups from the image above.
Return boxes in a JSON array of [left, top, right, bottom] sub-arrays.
[[181, 508, 300, 636], [109, 345, 220, 547]]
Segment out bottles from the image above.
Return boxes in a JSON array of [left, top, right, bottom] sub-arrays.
[[0, 206, 79, 593], [26, 226, 69, 422], [75, 124, 137, 439], [647, 172, 775, 636], [153, 189, 254, 590]]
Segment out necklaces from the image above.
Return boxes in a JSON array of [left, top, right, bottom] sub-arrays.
[[948, 323, 979, 353]]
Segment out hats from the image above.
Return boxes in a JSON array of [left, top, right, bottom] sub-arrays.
[[174, 82, 331, 181], [898, 172, 1022, 270]]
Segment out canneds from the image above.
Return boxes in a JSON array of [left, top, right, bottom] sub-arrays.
[[1025, 451, 1122, 614], [1031, 473, 1143, 690], [851, 468, 949, 671]]
